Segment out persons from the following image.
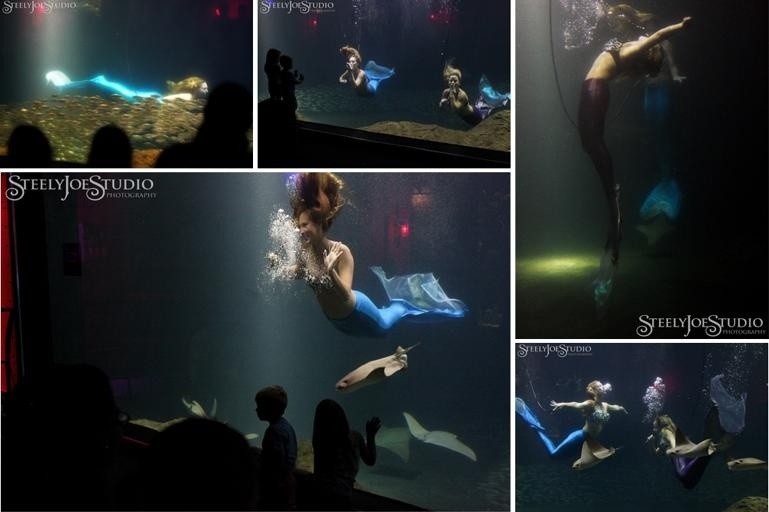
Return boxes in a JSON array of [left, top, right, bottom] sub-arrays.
[[47, 70, 208, 105], [645, 415, 720, 490], [312, 398, 381, 491], [338, 46, 397, 97], [515, 380, 629, 459], [578, 0, 692, 303], [5, 123, 53, 167], [264, 48, 304, 120], [439, 65, 509, 123], [255, 385, 298, 469], [151, 81, 253, 167], [265, 172, 470, 337], [85, 124, 133, 167]]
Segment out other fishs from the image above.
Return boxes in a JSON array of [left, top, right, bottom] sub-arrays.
[[571, 437, 622, 470], [334, 342, 422, 393], [364, 411, 477, 463], [659, 428, 722, 457], [181, 397, 217, 419], [726, 456, 768, 471], [0, 92, 208, 164], [711, 374, 746, 434]]
